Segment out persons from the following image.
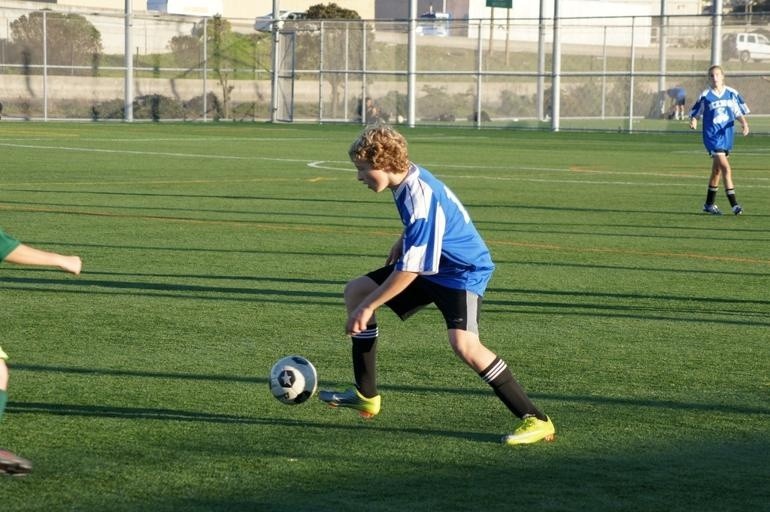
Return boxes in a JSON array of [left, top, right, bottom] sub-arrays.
[[0, 231, 83, 477], [666, 88, 686, 121], [688, 65, 751, 216], [317, 124, 557, 447], [356, 97, 393, 126], [542, 93, 552, 121], [645, 90, 667, 119]]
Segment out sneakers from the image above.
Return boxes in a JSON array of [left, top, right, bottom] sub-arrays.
[[703, 204, 721, 215], [501, 412, 556, 444], [731, 204, 742, 215], [318, 386, 380, 418], [0, 449, 32, 476]]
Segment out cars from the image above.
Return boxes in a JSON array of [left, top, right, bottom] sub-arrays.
[[252, 8, 309, 35], [400, 14, 451, 37], [721, 31, 770, 64]]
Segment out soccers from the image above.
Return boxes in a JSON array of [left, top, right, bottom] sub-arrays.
[[269, 356, 317, 404]]
